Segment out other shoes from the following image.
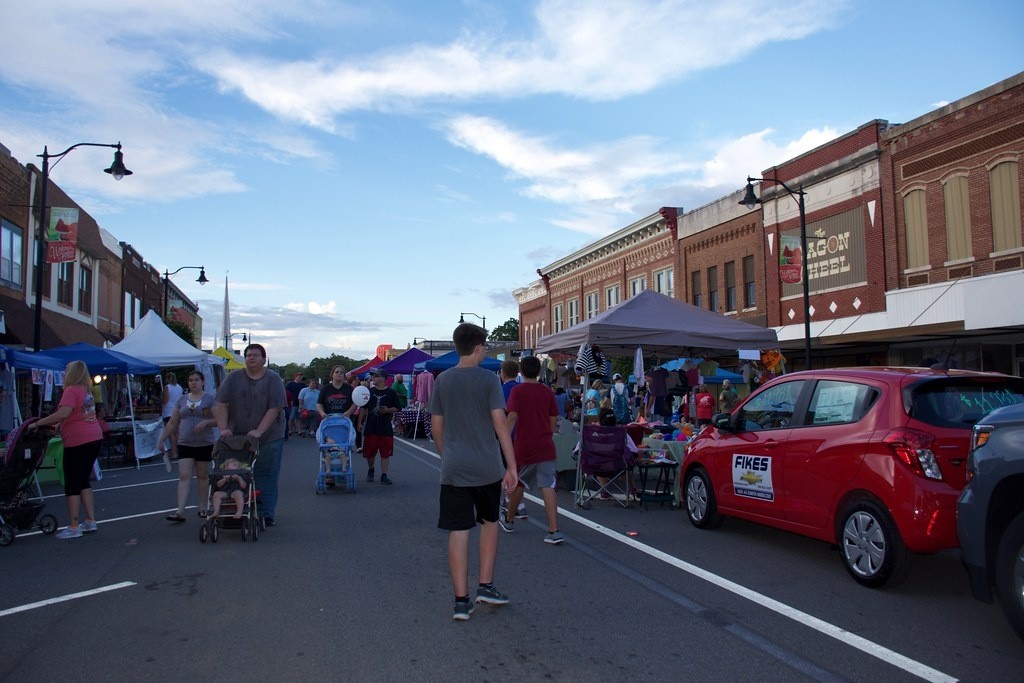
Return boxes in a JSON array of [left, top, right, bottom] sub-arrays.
[[601, 491, 612, 498], [367, 471, 374, 481], [381, 475, 392, 484], [166, 512, 186, 522], [199, 509, 207, 517], [629, 488, 641, 500], [265, 517, 275, 525], [502, 496, 528, 519], [168, 451, 177, 459]]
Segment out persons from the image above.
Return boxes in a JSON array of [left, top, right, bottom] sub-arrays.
[[28, 323, 741, 620]]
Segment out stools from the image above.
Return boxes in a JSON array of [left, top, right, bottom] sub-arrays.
[[633, 457, 683, 511]]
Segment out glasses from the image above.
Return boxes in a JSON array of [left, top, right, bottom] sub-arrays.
[[373, 375, 380, 377]]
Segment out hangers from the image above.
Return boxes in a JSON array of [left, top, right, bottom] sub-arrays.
[[537, 351, 574, 370], [684, 356, 713, 366], [589, 339, 603, 354], [424, 370, 428, 373], [635, 343, 644, 351]]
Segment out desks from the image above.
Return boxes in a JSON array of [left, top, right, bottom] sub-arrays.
[[102, 419, 170, 470], [642, 437, 689, 505], [392, 409, 426, 436]]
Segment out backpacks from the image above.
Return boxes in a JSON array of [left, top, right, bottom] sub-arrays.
[[612, 385, 632, 423]]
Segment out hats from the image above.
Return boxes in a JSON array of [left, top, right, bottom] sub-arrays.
[[302, 408, 308, 417], [295, 372, 303, 376], [613, 372, 621, 380], [370, 369, 387, 378]]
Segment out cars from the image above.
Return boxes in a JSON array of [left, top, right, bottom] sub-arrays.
[[953, 404, 1024, 646], [678, 365, 1024, 589]]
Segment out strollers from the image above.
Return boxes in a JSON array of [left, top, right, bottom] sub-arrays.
[[0, 417, 61, 546], [314, 413, 358, 494], [197, 433, 268, 542]]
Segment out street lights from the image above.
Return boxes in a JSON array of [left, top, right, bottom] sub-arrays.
[[161, 265, 211, 323], [412, 337, 433, 355], [32, 141, 135, 417], [224, 332, 248, 355], [736, 169, 814, 370], [458, 312, 487, 331]]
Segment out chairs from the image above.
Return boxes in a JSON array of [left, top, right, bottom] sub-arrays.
[[98, 435, 129, 465], [574, 422, 637, 511]]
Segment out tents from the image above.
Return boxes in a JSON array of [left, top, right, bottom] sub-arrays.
[[344, 287, 792, 508], [0, 309, 249, 470]]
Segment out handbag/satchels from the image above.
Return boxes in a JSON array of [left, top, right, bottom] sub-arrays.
[[565, 400, 571, 411]]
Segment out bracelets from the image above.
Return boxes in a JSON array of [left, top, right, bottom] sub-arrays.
[[36, 422, 38, 427]]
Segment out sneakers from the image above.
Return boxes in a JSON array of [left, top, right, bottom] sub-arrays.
[[499, 515, 514, 532], [80, 520, 98, 531], [453, 597, 475, 619], [543, 531, 564, 543], [474, 588, 510, 604], [56, 527, 82, 537]]
